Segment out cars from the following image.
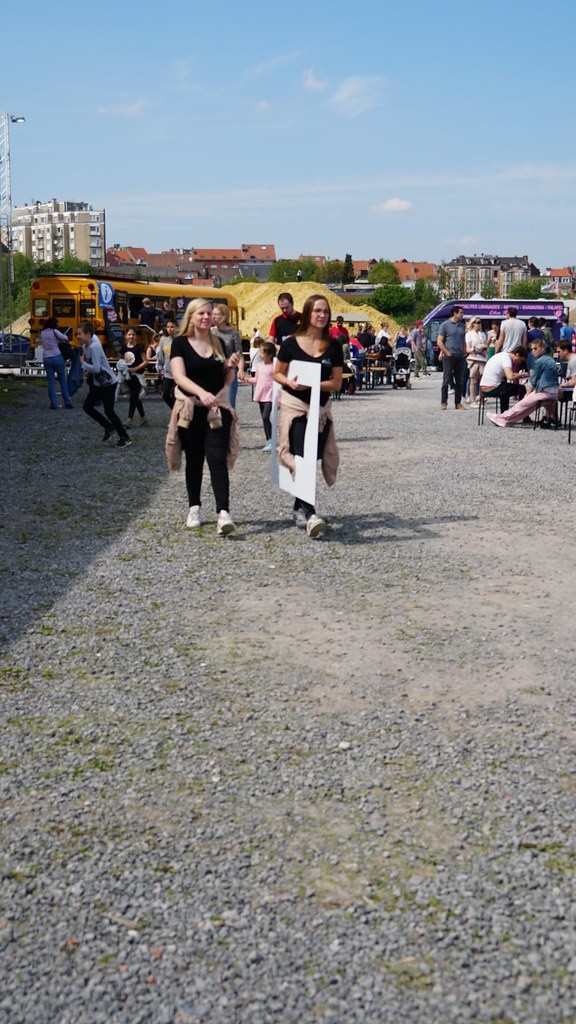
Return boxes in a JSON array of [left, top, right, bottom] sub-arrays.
[[0, 334, 35, 367]]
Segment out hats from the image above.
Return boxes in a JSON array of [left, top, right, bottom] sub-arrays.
[[416, 320, 423, 329]]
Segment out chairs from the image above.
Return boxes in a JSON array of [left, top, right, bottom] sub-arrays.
[[144, 351, 576, 444]]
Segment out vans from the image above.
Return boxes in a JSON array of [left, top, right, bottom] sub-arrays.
[[392, 299, 570, 372], [241, 335, 250, 370]]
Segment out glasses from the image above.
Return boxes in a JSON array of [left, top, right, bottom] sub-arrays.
[[529, 347, 538, 352], [383, 324, 389, 328], [473, 320, 482, 325]]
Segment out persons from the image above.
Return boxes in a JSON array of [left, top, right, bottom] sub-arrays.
[[556, 340, 576, 388], [155, 318, 180, 410], [273, 293, 344, 537], [160, 299, 176, 325], [146, 333, 162, 396], [495, 307, 527, 400], [169, 299, 240, 536], [329, 316, 431, 391], [76, 323, 132, 448], [480, 346, 533, 423], [486, 338, 559, 428], [465, 316, 492, 408], [119, 327, 148, 428], [210, 292, 302, 452], [297, 267, 302, 282], [437, 306, 466, 410], [526, 316, 575, 342], [487, 322, 499, 345], [41, 318, 74, 409], [138, 298, 159, 329]]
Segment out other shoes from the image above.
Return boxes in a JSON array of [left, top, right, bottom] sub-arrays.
[[306, 513, 325, 537], [341, 368, 520, 411], [216, 510, 236, 535], [64, 404, 75, 409], [50, 404, 57, 409], [522, 416, 535, 425], [187, 511, 202, 528], [137, 420, 149, 429], [292, 508, 307, 527], [486, 411, 507, 428], [539, 416, 563, 429], [262, 439, 273, 452], [102, 424, 116, 442], [121, 423, 131, 430], [114, 437, 133, 449]]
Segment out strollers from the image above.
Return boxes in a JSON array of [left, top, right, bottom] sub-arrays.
[[392, 347, 412, 390]]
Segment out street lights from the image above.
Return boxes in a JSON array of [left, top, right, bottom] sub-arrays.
[[0, 113, 26, 353]]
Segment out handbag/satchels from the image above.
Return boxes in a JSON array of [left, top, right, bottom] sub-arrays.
[[53, 329, 74, 361]]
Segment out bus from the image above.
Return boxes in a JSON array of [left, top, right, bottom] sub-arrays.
[[30, 272, 245, 373]]
